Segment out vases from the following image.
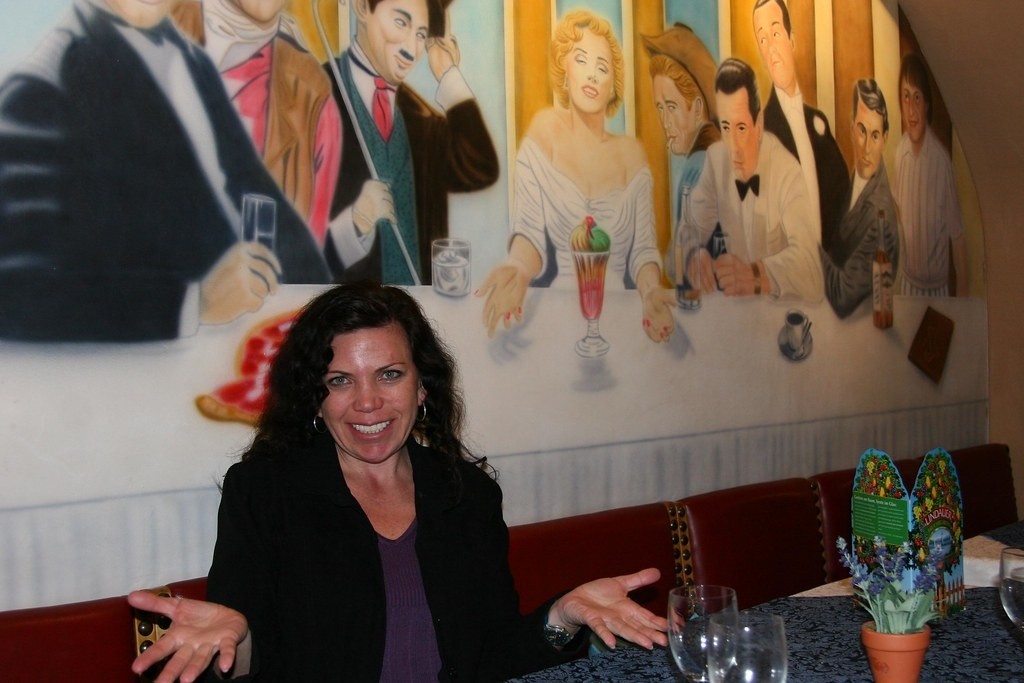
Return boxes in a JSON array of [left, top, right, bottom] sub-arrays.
[[860, 622, 932, 683]]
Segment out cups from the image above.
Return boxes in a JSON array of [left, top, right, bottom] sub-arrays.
[[707, 611, 788, 683], [667, 585, 738, 683], [998, 546, 1024, 632]]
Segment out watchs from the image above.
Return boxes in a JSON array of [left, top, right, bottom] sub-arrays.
[[543, 625, 580, 647]]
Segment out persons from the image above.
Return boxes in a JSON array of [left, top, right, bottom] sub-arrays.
[[128, 280, 670, 683]]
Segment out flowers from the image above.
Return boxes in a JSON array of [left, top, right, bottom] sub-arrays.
[[835, 534, 944, 634]]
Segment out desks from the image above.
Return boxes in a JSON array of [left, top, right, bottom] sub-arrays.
[[505, 518, 1024, 683]]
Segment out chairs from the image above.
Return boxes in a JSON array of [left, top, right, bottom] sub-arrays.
[[0, 442, 1024, 683]]
